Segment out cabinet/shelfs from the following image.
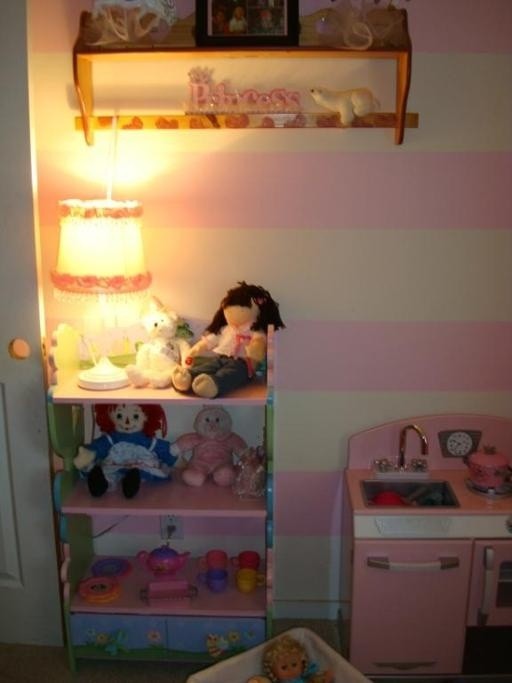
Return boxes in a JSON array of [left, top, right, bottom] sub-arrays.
[[43, 324, 277, 676], [350, 509, 512, 678]]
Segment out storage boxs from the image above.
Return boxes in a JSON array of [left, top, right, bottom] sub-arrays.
[[187, 624, 373, 683]]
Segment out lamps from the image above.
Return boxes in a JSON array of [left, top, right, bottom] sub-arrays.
[[49, 197, 153, 392]]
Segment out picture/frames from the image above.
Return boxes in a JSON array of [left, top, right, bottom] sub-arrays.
[[193, 0, 300, 47]]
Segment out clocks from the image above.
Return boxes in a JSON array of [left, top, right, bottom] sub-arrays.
[[438, 429, 481, 458]]
